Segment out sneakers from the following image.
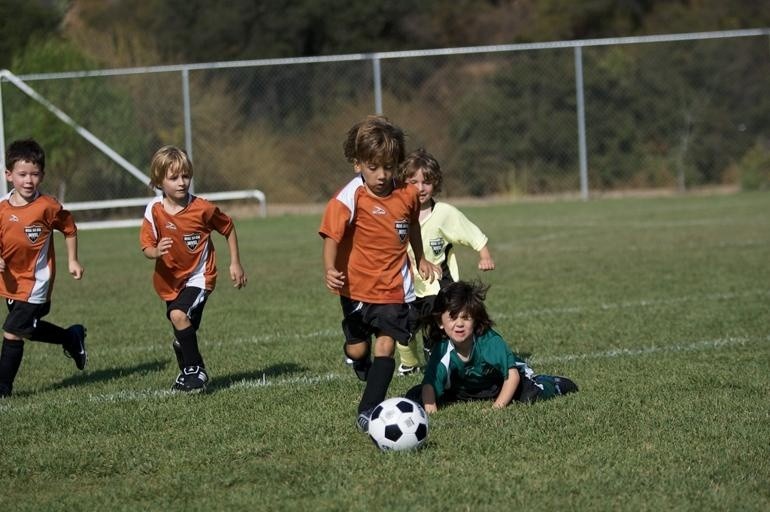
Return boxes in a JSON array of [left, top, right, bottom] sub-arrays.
[[354, 336, 373, 382], [171, 364, 209, 390], [62, 325, 86, 371], [533, 374, 579, 396], [399, 348, 431, 376], [356, 408, 375, 433]]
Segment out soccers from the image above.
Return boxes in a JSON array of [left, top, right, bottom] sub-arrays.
[[368, 397, 429, 453]]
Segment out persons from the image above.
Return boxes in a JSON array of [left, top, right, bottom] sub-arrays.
[[406, 278, 580, 417], [318, 114, 446, 437], [0, 141, 88, 397], [139, 146, 248, 394], [393, 147, 495, 378]]
